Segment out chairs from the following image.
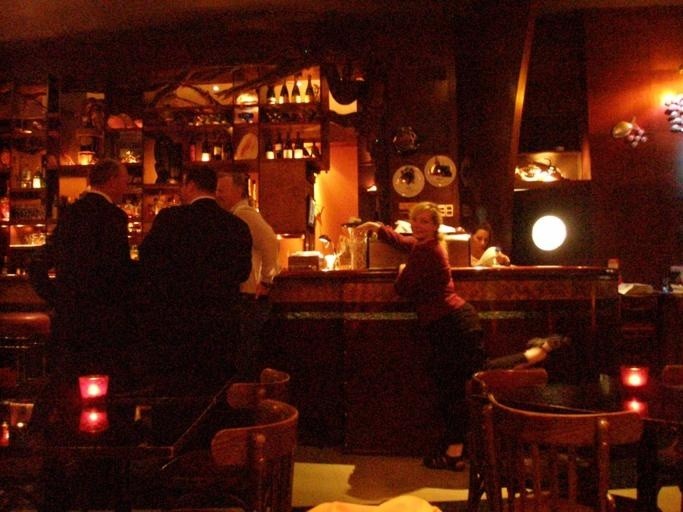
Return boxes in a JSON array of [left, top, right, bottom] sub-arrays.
[[465, 358, 644, 511], [173, 367, 300, 512]]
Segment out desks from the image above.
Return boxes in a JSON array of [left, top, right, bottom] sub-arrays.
[[478, 367, 682, 476], [21, 333, 261, 510]]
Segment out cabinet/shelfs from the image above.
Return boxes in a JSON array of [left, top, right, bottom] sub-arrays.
[[1, 66, 329, 291]]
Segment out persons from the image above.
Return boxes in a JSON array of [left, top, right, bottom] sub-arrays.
[[137, 162, 252, 445], [215, 170, 281, 357], [482, 315, 601, 490], [470, 225, 512, 267], [26, 152, 137, 384], [353, 200, 483, 473]]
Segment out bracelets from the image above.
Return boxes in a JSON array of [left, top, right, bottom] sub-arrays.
[[260, 281, 270, 289]]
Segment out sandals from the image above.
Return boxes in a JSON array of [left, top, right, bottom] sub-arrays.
[[423, 451, 466, 472]]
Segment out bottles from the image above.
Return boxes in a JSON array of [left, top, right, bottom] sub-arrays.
[[265, 74, 316, 104], [263, 128, 320, 159], [187, 132, 234, 163]]
